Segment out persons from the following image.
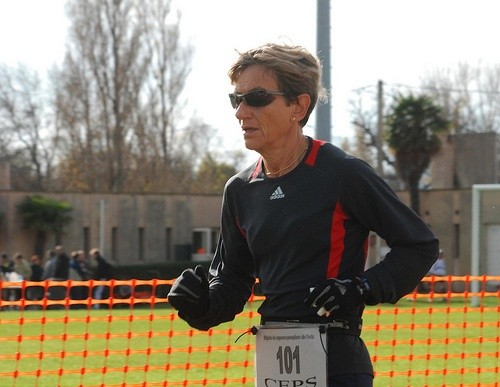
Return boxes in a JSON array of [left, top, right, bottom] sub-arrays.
[[166, 43, 438, 387], [0, 246, 120, 310]]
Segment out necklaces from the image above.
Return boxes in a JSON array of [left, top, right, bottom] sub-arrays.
[[264, 135, 309, 175]]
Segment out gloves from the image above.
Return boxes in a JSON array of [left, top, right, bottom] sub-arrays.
[[304, 277, 367, 318], [167, 265, 210, 322]]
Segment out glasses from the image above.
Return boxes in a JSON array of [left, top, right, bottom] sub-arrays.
[[229, 89, 289, 109]]
